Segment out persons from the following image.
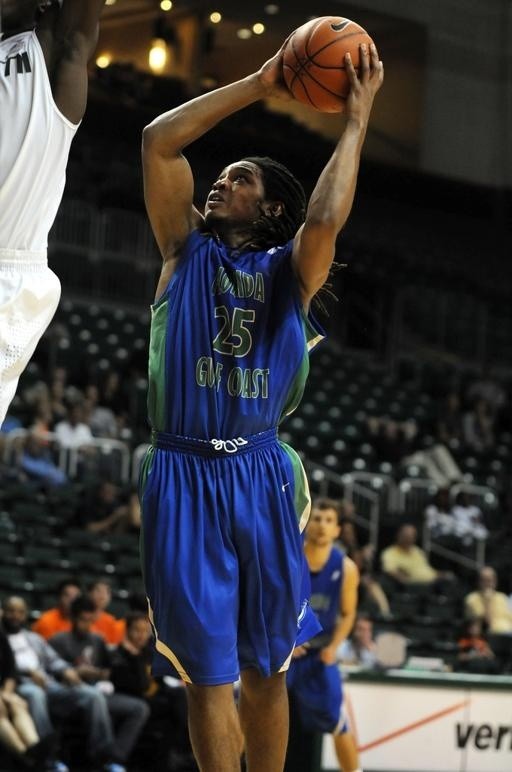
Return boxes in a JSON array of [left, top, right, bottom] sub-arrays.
[[287, 359, 512, 772], [136, 31, 388, 772], [0, 360, 201, 772], [0, 0, 108, 435]]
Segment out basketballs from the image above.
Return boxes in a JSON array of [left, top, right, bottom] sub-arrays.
[[283, 17, 380, 113]]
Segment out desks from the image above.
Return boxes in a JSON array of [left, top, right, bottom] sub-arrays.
[[320, 669, 512, 772]]
